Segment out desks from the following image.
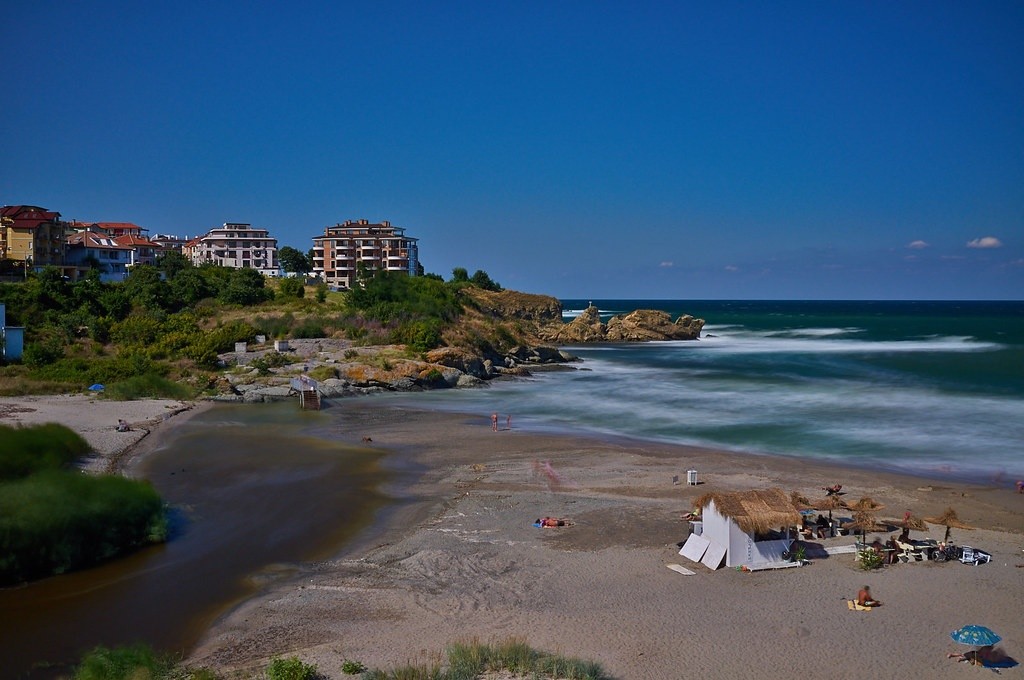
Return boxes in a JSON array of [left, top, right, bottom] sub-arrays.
[[861, 543, 895, 564], [807, 525, 820, 539], [899, 540, 933, 561]]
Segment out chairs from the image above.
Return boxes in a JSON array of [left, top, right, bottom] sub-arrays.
[[962, 545, 990, 566]]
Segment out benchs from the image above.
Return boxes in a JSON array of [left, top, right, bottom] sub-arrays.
[[854, 536, 946, 564], [798, 516, 844, 538]]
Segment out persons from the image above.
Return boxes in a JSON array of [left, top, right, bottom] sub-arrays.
[[886, 535, 901, 564], [859, 585, 884, 606], [938, 542, 945, 553], [506, 414, 511, 426], [685, 507, 700, 521], [947, 644, 1001, 665], [779, 525, 799, 539], [904, 509, 912, 522], [491, 412, 498, 432], [802, 515, 812, 539], [816, 514, 830, 539], [545, 517, 575, 526], [870, 537, 885, 560]]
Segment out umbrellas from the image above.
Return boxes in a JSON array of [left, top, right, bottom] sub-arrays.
[[947, 624, 1003, 665], [88, 384, 104, 391]]
